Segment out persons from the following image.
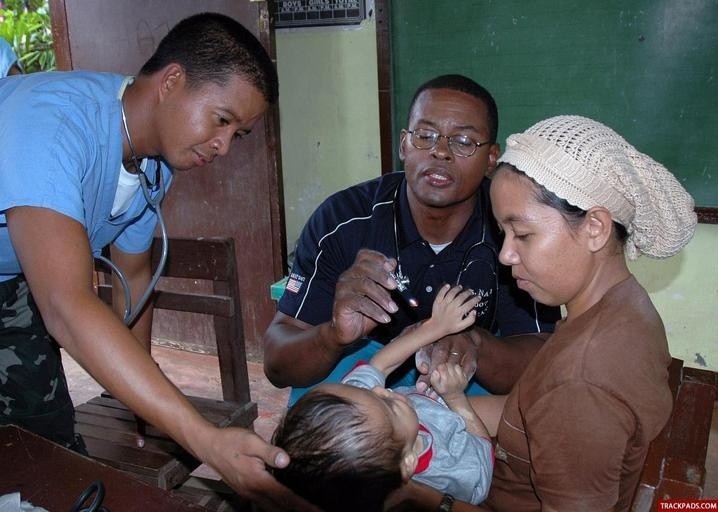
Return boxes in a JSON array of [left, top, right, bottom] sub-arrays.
[[268, 282, 511, 507], [1, 14, 317, 511], [0, 33, 23, 78], [263, 74, 560, 395], [381, 114, 697, 511]]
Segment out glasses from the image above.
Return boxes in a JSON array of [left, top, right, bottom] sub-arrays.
[[408, 126, 490, 157]]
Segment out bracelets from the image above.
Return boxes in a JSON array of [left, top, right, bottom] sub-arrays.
[[439, 493, 455, 511]]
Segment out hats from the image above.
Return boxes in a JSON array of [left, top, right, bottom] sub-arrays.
[[497, 116, 699, 261]]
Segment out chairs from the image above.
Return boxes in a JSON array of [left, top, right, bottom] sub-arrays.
[[71, 236, 259, 489], [629, 356, 718, 512]]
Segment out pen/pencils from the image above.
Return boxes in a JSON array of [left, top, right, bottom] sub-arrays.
[[383, 270, 418, 310]]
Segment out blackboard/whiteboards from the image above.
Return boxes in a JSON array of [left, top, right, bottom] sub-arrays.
[[375, 0, 718, 224]]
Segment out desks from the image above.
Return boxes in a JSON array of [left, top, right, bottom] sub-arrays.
[[0, 421, 205, 512]]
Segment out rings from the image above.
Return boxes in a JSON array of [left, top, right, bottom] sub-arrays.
[[448, 350, 461, 356]]
